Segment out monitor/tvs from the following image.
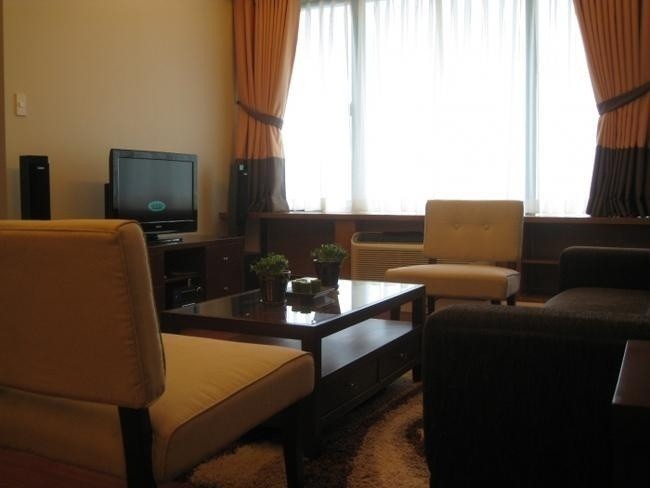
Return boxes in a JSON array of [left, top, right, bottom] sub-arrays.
[[105, 149, 197, 246]]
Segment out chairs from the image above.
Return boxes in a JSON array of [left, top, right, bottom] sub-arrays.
[[0, 218, 314, 486]]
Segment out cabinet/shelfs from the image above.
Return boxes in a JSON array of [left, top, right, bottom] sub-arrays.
[[146, 234, 245, 310]]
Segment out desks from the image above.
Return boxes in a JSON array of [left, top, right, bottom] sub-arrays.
[[612, 341, 650, 410]]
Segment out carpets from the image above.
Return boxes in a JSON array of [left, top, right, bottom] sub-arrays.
[[177, 297, 551, 486]]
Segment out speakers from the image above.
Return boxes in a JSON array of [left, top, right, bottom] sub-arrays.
[[19, 155, 51, 220]]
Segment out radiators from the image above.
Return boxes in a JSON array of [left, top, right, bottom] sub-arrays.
[[349, 229, 494, 301]]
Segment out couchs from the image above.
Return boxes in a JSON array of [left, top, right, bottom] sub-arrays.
[[423, 244, 650, 487], [384, 200, 525, 320]]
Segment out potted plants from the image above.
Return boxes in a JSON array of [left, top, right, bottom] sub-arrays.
[[308, 243, 350, 291], [249, 253, 292, 303]]
